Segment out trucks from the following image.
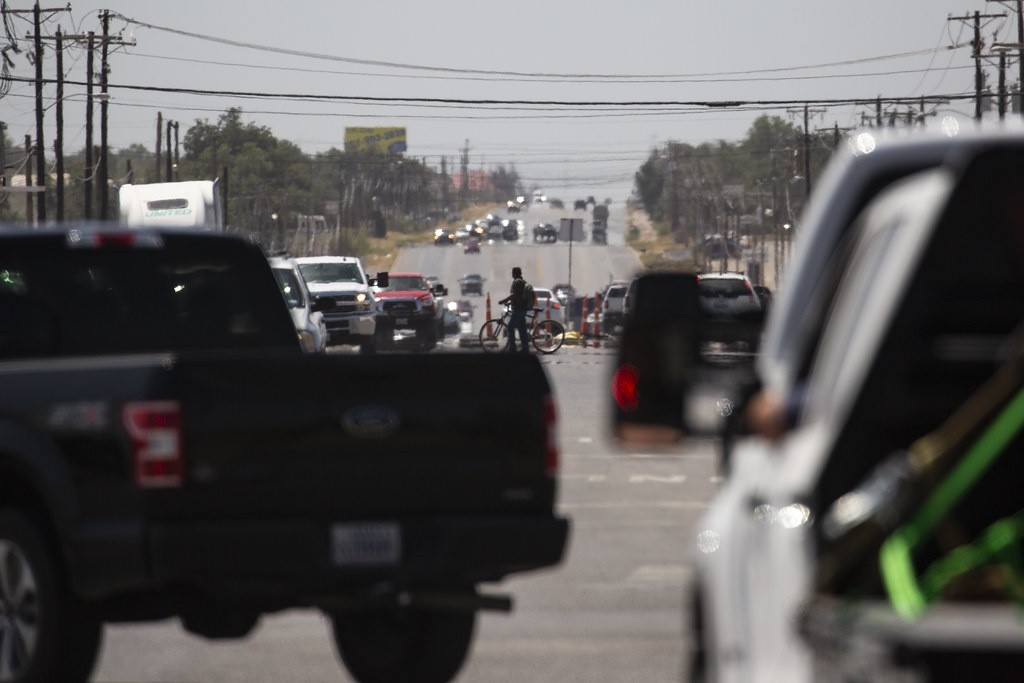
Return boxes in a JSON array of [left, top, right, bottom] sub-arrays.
[[118, 177, 224, 236]]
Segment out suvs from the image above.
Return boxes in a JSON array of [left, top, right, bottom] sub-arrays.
[[684, 117, 1024, 683], [262, 250, 462, 353], [457, 273, 487, 295], [585, 272, 772, 440]]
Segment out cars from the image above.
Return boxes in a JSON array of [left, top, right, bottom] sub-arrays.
[[533, 223, 558, 240], [506, 189, 562, 209], [433, 215, 519, 253], [503, 288, 565, 327], [449, 299, 478, 322]]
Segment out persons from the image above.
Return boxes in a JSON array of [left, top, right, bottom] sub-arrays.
[[498, 267, 530, 352]]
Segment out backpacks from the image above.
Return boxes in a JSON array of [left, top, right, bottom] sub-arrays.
[[521, 282, 535, 311]]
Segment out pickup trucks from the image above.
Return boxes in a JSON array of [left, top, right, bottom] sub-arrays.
[[0, 221, 574, 683]]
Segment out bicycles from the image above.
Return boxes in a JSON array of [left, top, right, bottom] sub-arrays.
[[478, 298, 566, 355]]
